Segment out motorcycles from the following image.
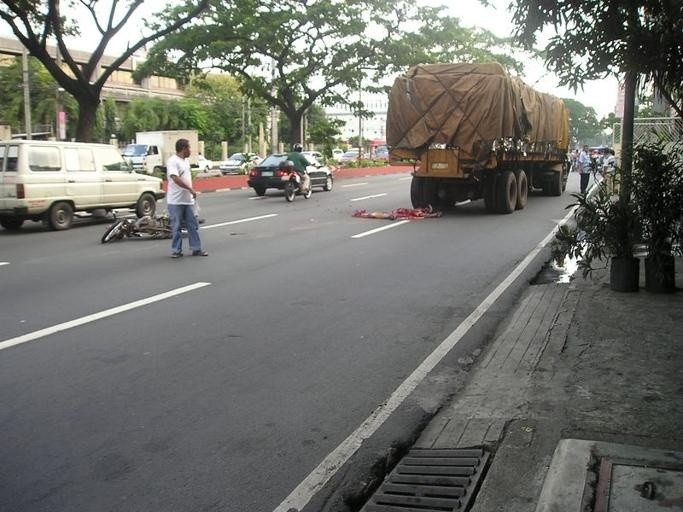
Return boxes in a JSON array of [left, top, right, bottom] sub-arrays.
[[278, 160, 313, 201], [101, 212, 207, 243]]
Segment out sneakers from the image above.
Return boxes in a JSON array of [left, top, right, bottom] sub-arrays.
[[171, 251, 183, 258], [192, 250, 208, 256]]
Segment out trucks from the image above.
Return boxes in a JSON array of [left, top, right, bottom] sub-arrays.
[[385, 60, 571, 213], [121, 129, 199, 176]]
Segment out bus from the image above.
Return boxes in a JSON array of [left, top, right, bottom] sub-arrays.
[[585, 147, 615, 170]]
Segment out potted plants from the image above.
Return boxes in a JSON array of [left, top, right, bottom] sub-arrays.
[[548, 135, 683, 294]]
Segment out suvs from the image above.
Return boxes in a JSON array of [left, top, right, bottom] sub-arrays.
[[1, 138, 166, 232]]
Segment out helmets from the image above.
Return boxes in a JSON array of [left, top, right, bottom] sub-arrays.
[[293, 143, 303, 152]]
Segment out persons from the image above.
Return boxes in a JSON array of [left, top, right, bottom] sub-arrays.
[[287, 143, 312, 195], [573, 148, 616, 172], [165, 138, 208, 258], [349, 201, 442, 222], [602, 148, 614, 196], [578, 144, 592, 194]]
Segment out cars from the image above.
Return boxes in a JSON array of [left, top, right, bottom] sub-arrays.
[[191, 154, 214, 174], [331, 145, 389, 166], [304, 150, 329, 162], [247, 152, 334, 196], [219, 151, 263, 175]]
